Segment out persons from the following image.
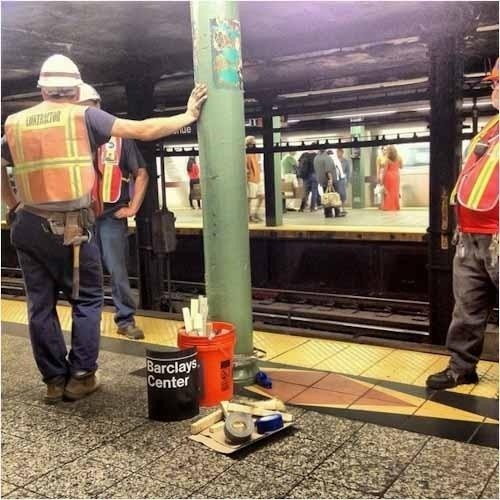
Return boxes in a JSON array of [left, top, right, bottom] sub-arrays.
[[77, 83, 150, 340], [426, 57, 499, 390], [186, 155, 201, 209], [245, 135, 264, 223], [1, 53, 209, 403], [377, 145, 387, 209], [281, 146, 352, 218], [382, 147, 403, 210]]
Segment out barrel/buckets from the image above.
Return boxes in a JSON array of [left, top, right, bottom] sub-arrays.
[[143, 345, 199, 422]]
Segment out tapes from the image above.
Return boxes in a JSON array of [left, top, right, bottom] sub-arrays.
[[224, 411, 255, 444], [256, 413, 284, 435]]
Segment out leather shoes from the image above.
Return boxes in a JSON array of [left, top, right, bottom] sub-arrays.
[[426, 368, 478, 390]]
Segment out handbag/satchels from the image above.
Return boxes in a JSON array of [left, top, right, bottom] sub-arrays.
[[321, 185, 340, 205]]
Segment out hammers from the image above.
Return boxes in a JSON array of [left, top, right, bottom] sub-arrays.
[[64, 235, 88, 301]]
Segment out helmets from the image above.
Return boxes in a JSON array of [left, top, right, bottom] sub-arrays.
[[37, 54, 101, 103]]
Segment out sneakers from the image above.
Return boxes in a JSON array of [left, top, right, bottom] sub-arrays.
[[64, 371, 96, 399], [118, 327, 144, 339], [44, 376, 66, 401]]
